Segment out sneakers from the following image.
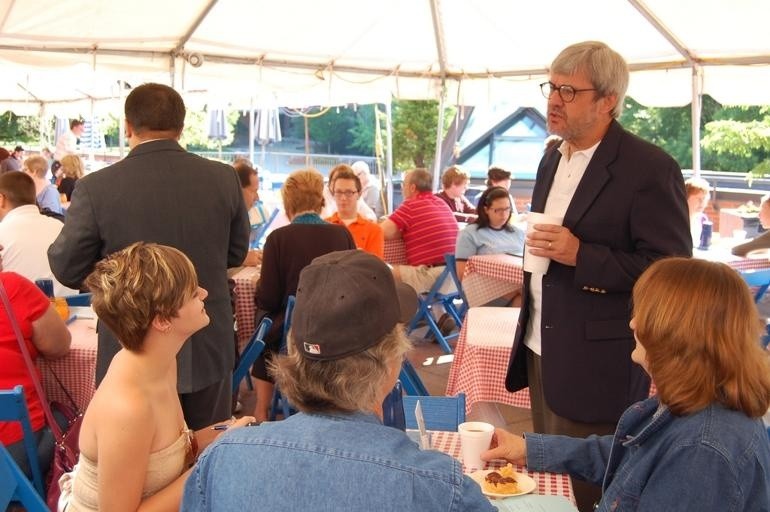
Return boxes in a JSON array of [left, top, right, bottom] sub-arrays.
[[416, 308, 436, 328], [430, 313, 456, 343]]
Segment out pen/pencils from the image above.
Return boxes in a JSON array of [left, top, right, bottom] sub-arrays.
[[211, 423, 259, 430], [64, 316, 76, 326]]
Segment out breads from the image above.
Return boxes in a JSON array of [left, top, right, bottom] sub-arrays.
[[483, 462, 520, 493]]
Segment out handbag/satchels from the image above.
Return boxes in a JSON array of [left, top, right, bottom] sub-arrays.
[[44, 412, 84, 512], [256, 304, 287, 348]]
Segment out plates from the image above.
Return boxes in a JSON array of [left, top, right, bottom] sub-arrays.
[[467, 470, 538, 498]]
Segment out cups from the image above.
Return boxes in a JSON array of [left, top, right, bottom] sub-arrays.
[[519, 209, 565, 280], [457, 419, 496, 471], [733, 228, 748, 242]]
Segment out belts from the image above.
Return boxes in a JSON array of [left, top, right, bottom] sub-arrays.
[[428, 263, 447, 268]]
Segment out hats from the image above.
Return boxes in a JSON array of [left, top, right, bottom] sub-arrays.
[[292, 249, 418, 361]]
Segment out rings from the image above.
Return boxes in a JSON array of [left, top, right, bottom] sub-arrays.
[[547, 240, 553, 251]]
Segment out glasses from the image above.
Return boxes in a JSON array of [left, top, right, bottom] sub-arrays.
[[540, 82, 596, 103], [488, 206, 514, 213], [332, 191, 358, 197]]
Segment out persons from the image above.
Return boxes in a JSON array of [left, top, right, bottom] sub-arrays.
[[0, 113, 770, 498], [181, 249, 493, 512], [480, 258, 770, 511], [57, 243, 258, 512], [519, 41, 691, 511], [47, 83, 252, 459]]
